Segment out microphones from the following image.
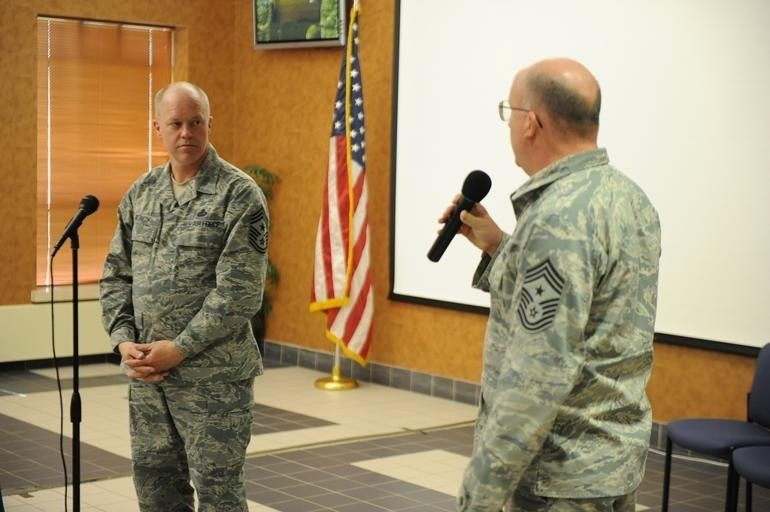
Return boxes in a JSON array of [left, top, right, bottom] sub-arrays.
[[50, 194, 99, 258], [428, 170, 492, 262]]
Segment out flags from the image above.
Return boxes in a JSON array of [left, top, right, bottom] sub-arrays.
[[310, 4, 374, 367]]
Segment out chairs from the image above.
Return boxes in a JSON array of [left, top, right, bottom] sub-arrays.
[[662, 343, 770, 512], [730, 445, 770, 512]]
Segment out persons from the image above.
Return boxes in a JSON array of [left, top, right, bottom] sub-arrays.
[[100, 81, 270, 512], [456, 57, 661, 512]]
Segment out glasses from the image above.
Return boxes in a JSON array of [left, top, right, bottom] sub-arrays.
[[497, 99, 543, 129]]
